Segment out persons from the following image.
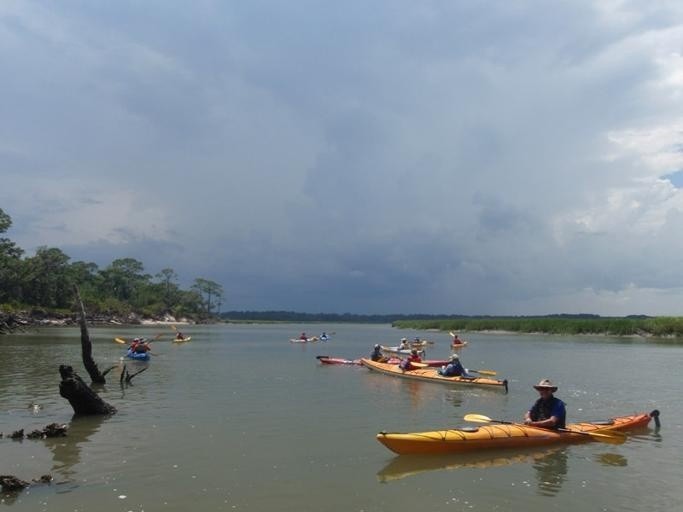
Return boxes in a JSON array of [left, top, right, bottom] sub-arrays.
[[370, 334, 464, 378], [319, 331, 330, 339], [523, 379, 567, 430], [127, 337, 152, 355], [176, 331, 185, 340], [300, 332, 307, 341]]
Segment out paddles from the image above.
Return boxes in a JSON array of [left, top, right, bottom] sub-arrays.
[[410, 362, 496, 376], [396, 356, 428, 367], [463, 413, 611, 438], [114, 338, 131, 345], [171, 325, 179, 332]]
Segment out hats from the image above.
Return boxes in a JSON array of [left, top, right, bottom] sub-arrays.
[[132, 336, 147, 345], [533, 378, 558, 392]]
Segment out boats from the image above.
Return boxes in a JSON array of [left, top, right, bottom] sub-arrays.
[[173, 336, 193, 343], [320, 358, 361, 367], [359, 340, 658, 454], [290, 333, 329, 344], [128, 349, 150, 359]]
